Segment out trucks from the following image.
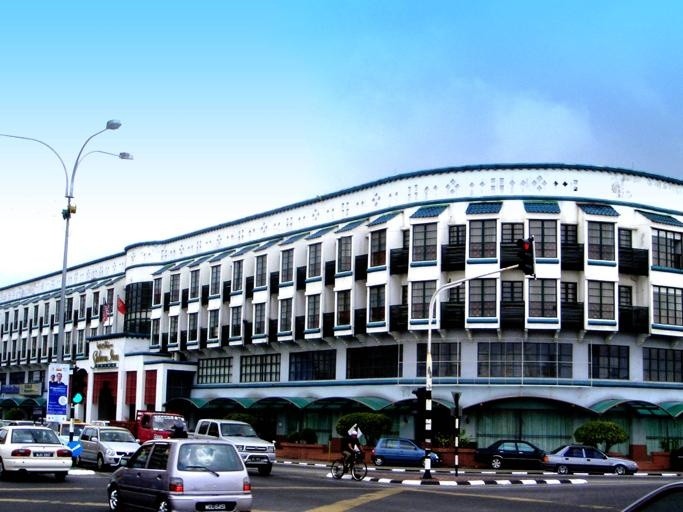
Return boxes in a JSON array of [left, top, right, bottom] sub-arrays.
[[108, 410, 188, 443]]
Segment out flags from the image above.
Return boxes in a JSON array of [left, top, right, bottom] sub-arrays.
[[116, 296, 127, 315], [99, 296, 110, 326]]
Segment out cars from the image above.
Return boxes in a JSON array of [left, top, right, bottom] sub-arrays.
[[372, 437, 440, 466], [542, 443, 639, 476], [477, 440, 548, 468], [103, 436, 254, 510], [0, 420, 138, 478]]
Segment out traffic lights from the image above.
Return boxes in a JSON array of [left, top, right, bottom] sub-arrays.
[[515, 237, 535, 275], [72, 369, 88, 403]]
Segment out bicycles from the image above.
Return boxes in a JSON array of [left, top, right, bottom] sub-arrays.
[[330, 449, 368, 479]]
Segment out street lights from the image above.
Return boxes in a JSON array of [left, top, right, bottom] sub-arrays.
[[0, 119, 134, 366]]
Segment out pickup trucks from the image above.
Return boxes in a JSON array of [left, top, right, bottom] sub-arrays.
[[185, 418, 278, 475]]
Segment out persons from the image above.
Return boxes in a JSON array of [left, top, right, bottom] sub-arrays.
[[52, 372, 66, 387], [339, 430, 363, 474], [48, 373, 56, 386], [167, 420, 189, 438]]
